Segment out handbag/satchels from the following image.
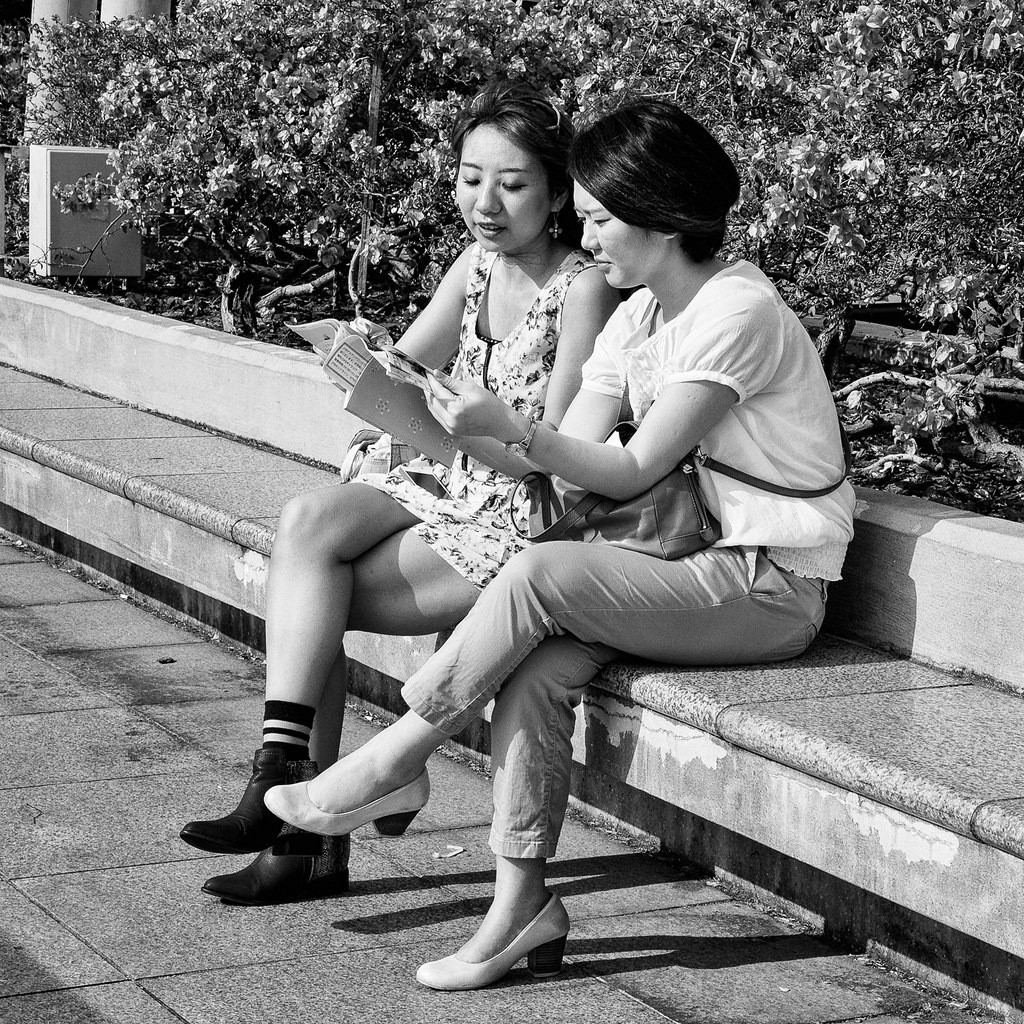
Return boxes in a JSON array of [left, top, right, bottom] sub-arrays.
[[340, 429, 422, 485], [510, 417, 726, 562]]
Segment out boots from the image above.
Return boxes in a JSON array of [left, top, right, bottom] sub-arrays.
[[180, 748, 309, 854], [202, 760, 350, 904]]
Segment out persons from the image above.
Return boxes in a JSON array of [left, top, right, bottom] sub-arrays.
[[259, 97, 859, 994], [178, 99, 622, 907]]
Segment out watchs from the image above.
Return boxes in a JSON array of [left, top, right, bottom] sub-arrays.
[[504, 417, 537, 457]]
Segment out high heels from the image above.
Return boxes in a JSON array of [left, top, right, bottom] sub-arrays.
[[416, 889, 570, 991], [263, 749, 430, 838]]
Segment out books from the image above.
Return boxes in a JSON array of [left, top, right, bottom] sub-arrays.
[[279, 304, 460, 468]]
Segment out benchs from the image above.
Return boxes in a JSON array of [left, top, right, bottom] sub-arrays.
[[0, 277, 1024, 1014]]
